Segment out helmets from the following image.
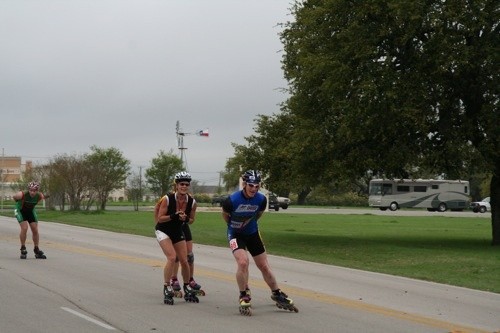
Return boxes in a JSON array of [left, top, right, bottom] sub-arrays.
[[242, 169, 262, 183], [175, 171, 192, 182], [29, 182, 40, 191]]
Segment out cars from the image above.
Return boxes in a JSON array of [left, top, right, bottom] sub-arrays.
[[269, 192, 279, 211], [211, 194, 229, 207], [273, 193, 291, 209], [470, 197, 491, 213]]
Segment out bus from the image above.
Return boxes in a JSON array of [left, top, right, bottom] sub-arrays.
[[368, 178, 470, 211]]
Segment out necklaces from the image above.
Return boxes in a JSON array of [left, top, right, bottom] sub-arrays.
[[175, 194, 186, 213]]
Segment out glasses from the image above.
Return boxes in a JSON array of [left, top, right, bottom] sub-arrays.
[[178, 182, 191, 186], [246, 183, 261, 188]]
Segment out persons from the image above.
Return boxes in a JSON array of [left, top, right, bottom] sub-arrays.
[[222, 169, 298, 316], [13, 182, 47, 259], [153, 171, 206, 305]]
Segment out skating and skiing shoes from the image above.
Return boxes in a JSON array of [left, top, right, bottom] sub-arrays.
[[189, 281, 205, 296], [172, 279, 183, 299], [163, 283, 175, 305], [270, 292, 299, 313], [20, 246, 27, 259], [34, 248, 47, 259], [238, 292, 253, 317], [183, 284, 200, 303]]
